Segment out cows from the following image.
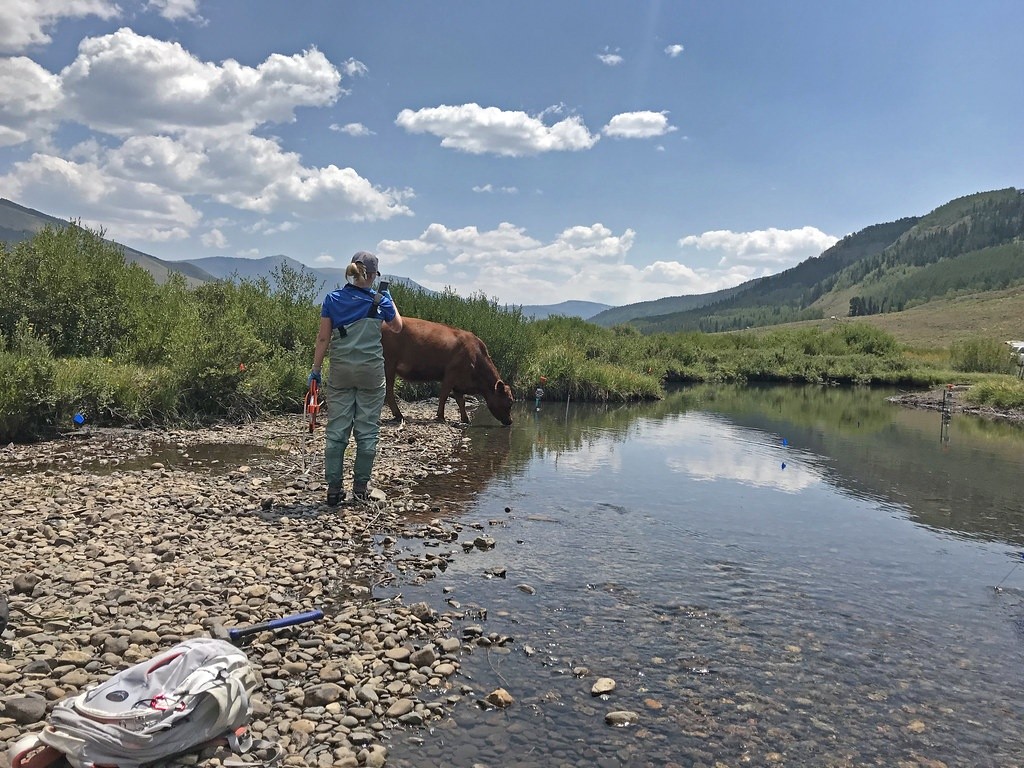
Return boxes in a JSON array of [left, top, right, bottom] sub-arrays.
[[381, 315, 514, 426]]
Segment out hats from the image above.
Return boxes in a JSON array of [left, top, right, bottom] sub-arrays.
[[351, 251, 380, 277]]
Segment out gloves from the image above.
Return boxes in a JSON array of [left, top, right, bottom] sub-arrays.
[[381, 289, 393, 301], [308, 372, 321, 385]]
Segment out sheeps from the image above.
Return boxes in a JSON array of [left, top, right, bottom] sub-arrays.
[[1007, 350, 1024, 380]]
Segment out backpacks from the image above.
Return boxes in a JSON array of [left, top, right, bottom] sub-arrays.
[[7, 637, 282, 768]]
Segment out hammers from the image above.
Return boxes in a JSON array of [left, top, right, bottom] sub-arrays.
[[209, 611, 323, 643]]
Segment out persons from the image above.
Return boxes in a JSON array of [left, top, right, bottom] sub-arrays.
[[306, 251, 403, 506]]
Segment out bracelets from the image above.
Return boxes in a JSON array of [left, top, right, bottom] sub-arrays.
[[312, 364, 323, 370]]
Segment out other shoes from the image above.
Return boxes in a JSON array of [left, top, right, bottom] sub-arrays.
[[354, 490, 368, 500], [327, 489, 345, 505]]
